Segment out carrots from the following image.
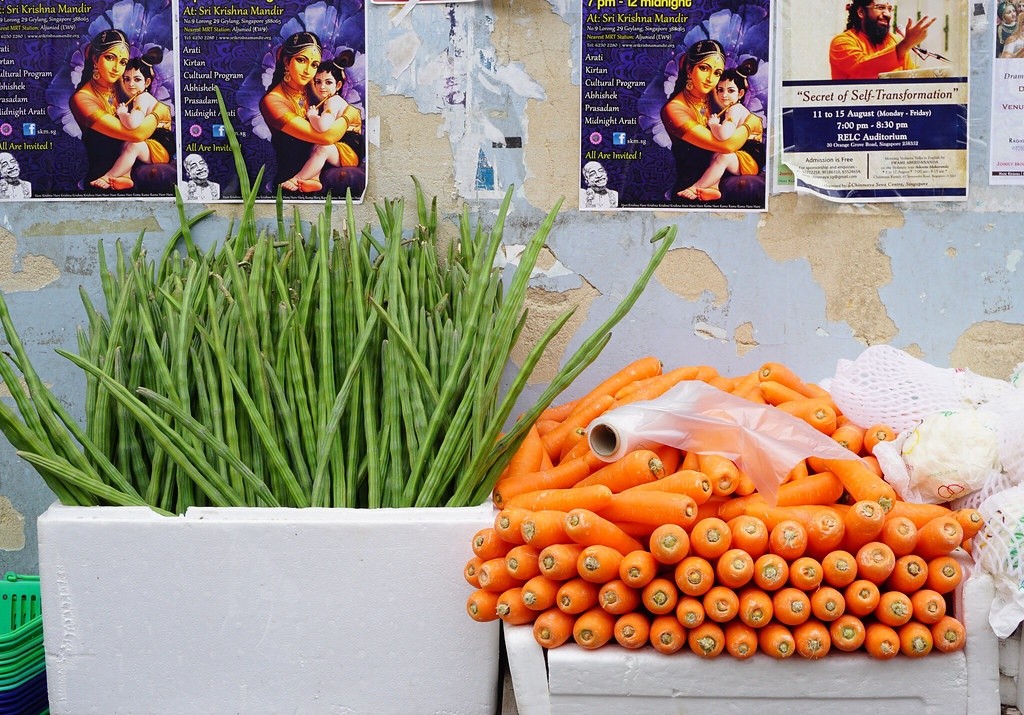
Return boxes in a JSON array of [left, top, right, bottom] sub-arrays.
[[463, 357, 985, 658]]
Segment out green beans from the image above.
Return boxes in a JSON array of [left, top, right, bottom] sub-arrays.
[[0, 86, 678, 518]]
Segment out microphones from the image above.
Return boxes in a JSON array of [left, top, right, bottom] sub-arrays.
[[896, 24, 919, 55]]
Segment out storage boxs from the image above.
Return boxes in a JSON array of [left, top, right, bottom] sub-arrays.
[[35, 474, 502, 715]]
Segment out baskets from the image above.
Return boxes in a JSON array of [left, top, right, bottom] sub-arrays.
[[0, 570, 50, 715]]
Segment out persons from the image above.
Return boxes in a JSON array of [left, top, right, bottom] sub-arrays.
[[828, 0, 937, 79]]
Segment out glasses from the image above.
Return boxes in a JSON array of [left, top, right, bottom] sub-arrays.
[[865, 5, 893, 13]]
